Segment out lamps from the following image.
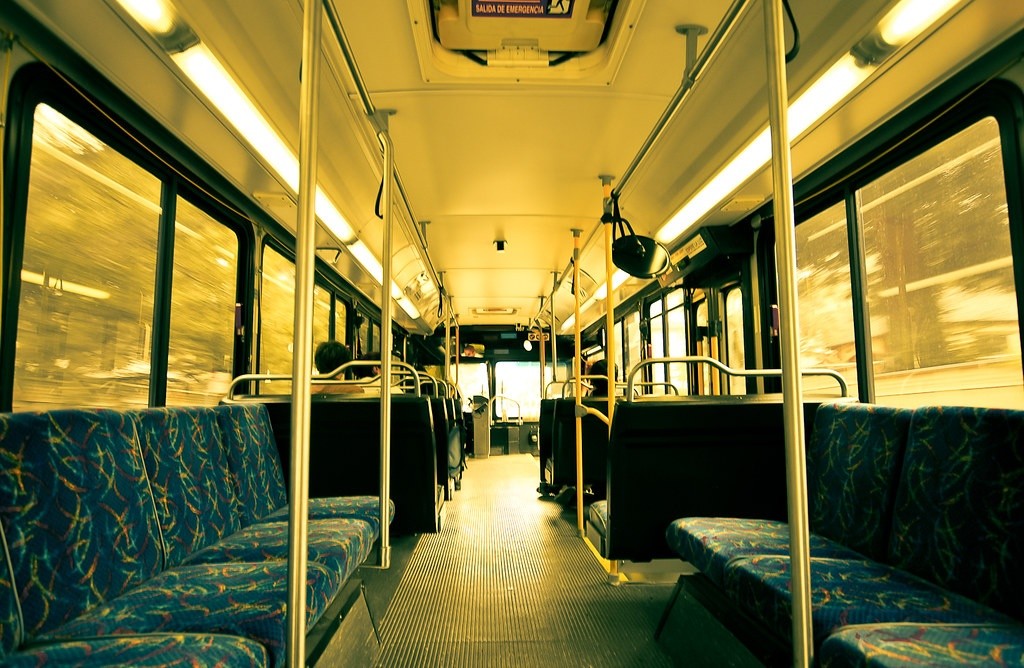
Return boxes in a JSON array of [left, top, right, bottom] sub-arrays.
[[108, 0, 438, 338], [558, 0, 964, 335]]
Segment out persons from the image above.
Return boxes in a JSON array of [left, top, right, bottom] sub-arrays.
[[311, 341, 364, 393], [460, 345, 483, 357], [352, 353, 404, 394], [581, 360, 618, 397]]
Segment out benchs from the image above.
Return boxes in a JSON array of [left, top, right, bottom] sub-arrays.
[[531, 351, 1024, 668], [0, 358, 469, 668]]
